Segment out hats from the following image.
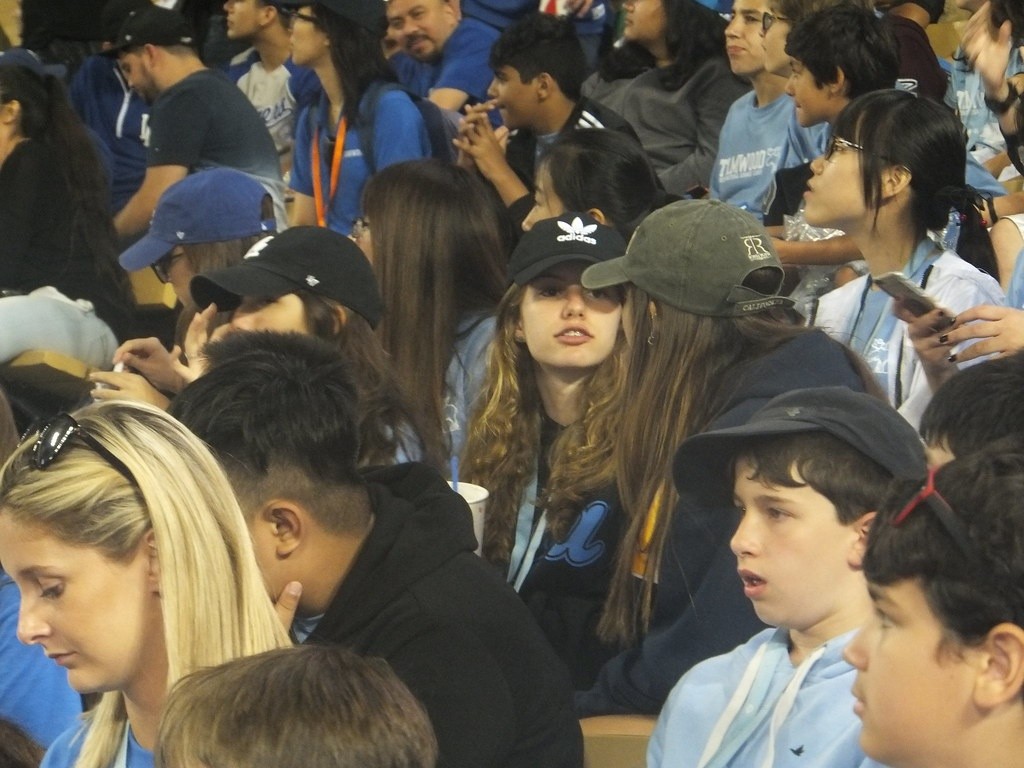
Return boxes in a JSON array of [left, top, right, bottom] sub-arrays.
[[118, 165, 278, 270], [0, 45, 68, 78], [190, 226, 383, 331], [582, 197, 795, 315], [101, 6, 193, 57], [505, 210, 626, 291], [670, 386, 930, 506], [323, 0, 387, 36]]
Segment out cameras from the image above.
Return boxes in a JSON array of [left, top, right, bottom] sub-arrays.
[[114, 361, 135, 375]]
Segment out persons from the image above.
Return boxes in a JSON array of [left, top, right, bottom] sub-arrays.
[[455, 24, 660, 223], [647, 386, 927, 768], [469, 214, 643, 604], [844, 436, 1024, 768], [798, 90, 1001, 439], [0, 0, 1024, 768], [581, 198, 889, 705], [521, 134, 665, 261], [0, 400, 268, 768], [160, 348, 586, 768]]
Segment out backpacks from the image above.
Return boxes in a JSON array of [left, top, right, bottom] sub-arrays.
[[308, 80, 468, 173]]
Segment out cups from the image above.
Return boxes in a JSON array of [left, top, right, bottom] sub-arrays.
[[445, 477, 487, 561], [95, 367, 130, 402]]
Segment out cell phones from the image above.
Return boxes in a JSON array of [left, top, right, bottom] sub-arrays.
[[873, 272, 955, 331]]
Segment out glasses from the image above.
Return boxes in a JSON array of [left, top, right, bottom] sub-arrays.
[[151, 246, 190, 285], [762, 12, 794, 30], [18, 411, 142, 490], [885, 461, 982, 575], [282, 8, 320, 28], [826, 135, 897, 165]]
